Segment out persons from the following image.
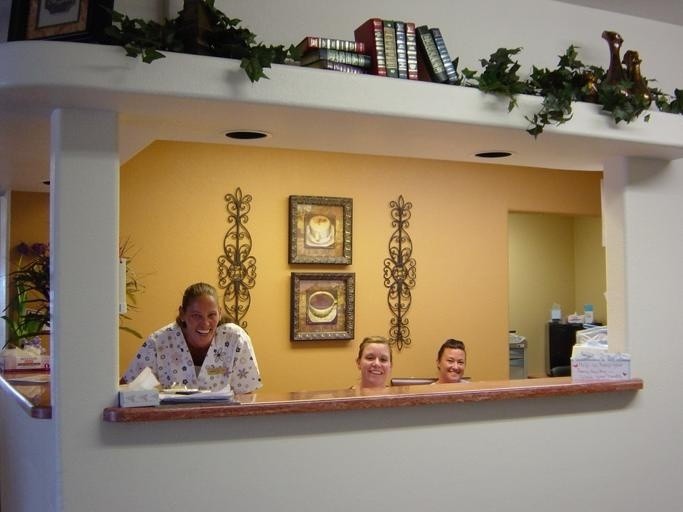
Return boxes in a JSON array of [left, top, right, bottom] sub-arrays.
[[119, 282, 263, 401], [429, 338, 473, 386], [345, 335, 394, 390]]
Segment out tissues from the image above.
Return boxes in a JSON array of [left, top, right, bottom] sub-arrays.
[[120, 367, 159, 408]]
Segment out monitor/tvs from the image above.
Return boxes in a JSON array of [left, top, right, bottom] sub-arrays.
[[548, 321, 602, 376]]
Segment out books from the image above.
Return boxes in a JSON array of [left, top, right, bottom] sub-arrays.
[[289, 36, 367, 60], [404, 22, 420, 81], [382, 20, 398, 79], [352, 18, 386, 77], [428, 26, 458, 84], [414, 23, 447, 83], [394, 20, 408, 79], [303, 61, 369, 75], [299, 50, 372, 67]]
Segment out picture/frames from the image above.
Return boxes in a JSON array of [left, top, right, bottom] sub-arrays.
[[285, 193, 357, 343], [5, 1, 117, 41]]
